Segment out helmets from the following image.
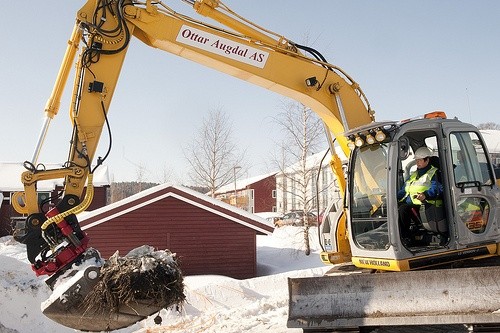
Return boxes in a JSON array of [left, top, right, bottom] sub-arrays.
[[413, 147, 430, 160]]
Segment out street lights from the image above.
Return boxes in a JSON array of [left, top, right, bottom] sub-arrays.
[[233, 166, 242, 206]]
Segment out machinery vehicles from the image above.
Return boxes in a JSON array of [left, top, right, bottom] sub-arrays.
[[11, 0, 500, 333]]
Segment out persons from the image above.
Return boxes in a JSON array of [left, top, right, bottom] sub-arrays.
[[396, 146, 445, 248]]
